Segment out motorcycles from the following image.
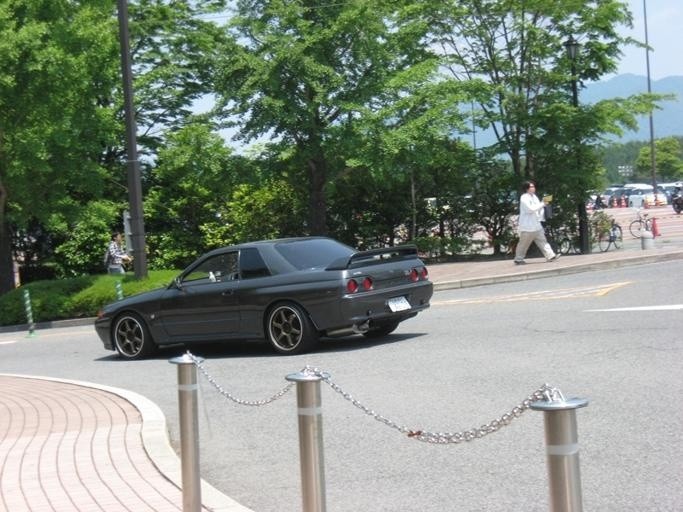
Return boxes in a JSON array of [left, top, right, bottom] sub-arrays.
[[672, 194, 683, 213]]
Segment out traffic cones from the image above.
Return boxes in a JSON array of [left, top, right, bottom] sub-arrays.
[[613, 194, 626, 208], [645, 198, 660, 209], [652, 217, 661, 237]]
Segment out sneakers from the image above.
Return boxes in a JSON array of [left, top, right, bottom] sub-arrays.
[[514, 261, 525, 265], [548, 253, 561, 261]]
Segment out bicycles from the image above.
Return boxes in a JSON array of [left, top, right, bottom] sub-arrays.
[[559, 211, 655, 255]]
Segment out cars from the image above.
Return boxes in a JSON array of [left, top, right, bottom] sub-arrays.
[[586, 181, 683, 206], [94, 235, 434, 360]]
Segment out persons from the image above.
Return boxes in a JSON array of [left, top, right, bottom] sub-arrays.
[[104, 230, 131, 275], [595, 195, 603, 209], [512, 180, 561, 266]]
